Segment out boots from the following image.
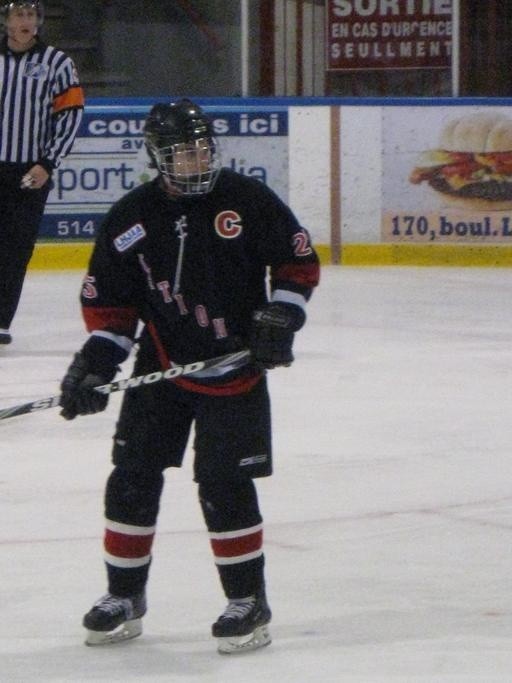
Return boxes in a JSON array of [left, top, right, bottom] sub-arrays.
[[211, 577, 271, 638], [81, 585, 148, 632]]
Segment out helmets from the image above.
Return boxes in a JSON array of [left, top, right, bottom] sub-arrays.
[[145, 99, 224, 194], [0, 0, 46, 28]]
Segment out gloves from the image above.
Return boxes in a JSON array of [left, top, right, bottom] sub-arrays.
[[60, 351, 120, 420], [247, 303, 304, 368]]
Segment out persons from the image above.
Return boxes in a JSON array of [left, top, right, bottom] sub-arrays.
[[1, 0, 86, 342], [60, 94, 320, 636]]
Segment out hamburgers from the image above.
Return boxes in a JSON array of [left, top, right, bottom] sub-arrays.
[[409, 111, 512, 210]]
